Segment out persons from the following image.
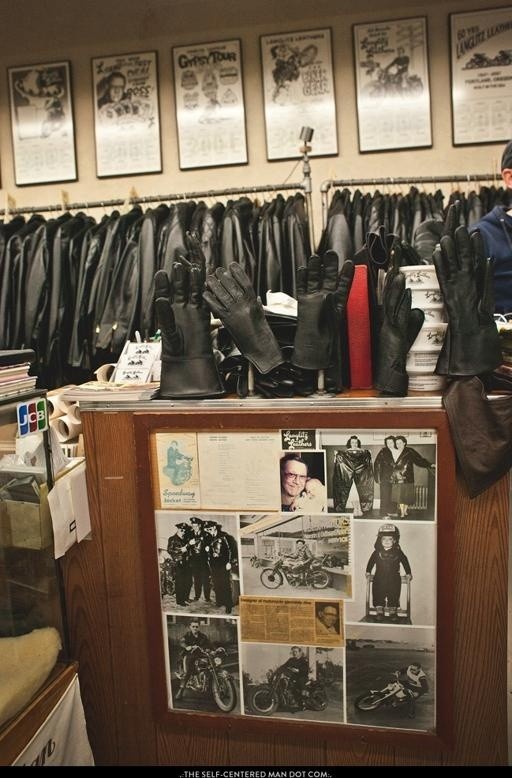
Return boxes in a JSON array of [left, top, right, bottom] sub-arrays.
[[166, 517, 239, 704], [384, 47, 410, 89], [464, 140, 512, 314], [270, 434, 436, 718], [95, 72, 134, 118]]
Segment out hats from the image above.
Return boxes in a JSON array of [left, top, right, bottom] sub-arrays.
[[203, 521, 217, 528], [175, 523, 188, 529], [374, 524, 399, 549], [190, 517, 203, 524]]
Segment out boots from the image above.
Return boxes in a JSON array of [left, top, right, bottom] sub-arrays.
[[175, 687, 184, 699]]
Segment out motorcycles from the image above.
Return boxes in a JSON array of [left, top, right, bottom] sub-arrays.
[[255, 551, 333, 594], [159, 555, 175, 600], [176, 650, 242, 712], [247, 663, 331, 716], [354, 669, 438, 716]]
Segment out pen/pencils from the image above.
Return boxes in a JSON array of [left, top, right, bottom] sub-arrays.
[[135, 329, 162, 343]]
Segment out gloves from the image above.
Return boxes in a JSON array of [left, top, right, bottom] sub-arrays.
[[154, 262, 226, 399], [179, 230, 206, 281], [377, 266, 423, 397], [432, 224, 505, 378], [202, 262, 286, 373], [291, 251, 354, 370], [217, 345, 342, 398]]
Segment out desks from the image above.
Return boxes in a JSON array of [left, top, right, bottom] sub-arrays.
[[60, 410, 511, 767]]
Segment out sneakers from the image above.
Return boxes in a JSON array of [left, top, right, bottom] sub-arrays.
[[376, 614, 385, 621], [390, 614, 400, 621]]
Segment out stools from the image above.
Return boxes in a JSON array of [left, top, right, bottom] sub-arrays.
[[1, 660, 97, 766]]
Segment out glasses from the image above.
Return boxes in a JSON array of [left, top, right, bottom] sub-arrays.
[[282, 470, 309, 482]]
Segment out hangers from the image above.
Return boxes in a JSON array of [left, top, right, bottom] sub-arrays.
[[0, 183, 300, 226], [328, 173, 492, 196]]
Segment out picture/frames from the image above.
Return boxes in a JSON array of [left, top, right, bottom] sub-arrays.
[[131, 409, 457, 753]]
